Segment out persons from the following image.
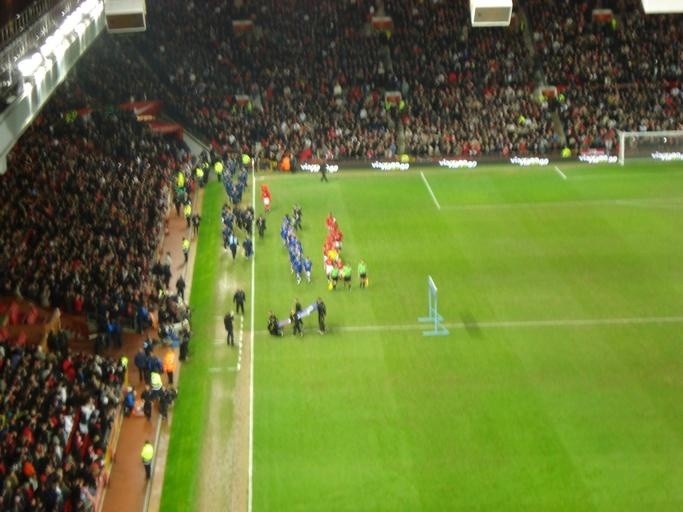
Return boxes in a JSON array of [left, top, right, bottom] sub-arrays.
[[318, 160, 328, 182], [268, 205, 367, 337], [0, 1, 683, 172], [0, 168, 273, 512]]
